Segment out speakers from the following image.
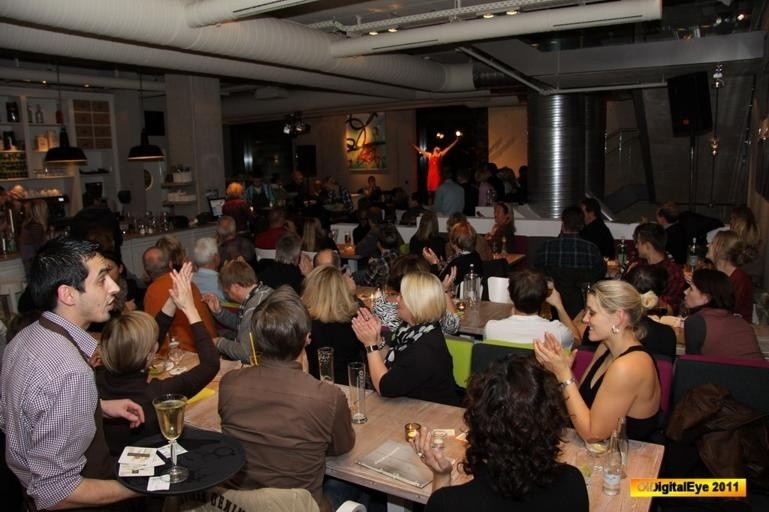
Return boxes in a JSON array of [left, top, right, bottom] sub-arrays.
[[666, 71, 713, 137], [295, 144, 317, 178]]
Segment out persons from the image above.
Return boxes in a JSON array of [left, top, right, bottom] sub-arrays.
[[0, 172, 766, 512], [409, 134, 459, 207]]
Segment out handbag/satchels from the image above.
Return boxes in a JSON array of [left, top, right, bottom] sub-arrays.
[[665, 381, 768, 478]]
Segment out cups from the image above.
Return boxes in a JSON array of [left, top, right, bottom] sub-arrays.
[[346, 362, 368, 425], [582, 435, 610, 457], [575, 449, 597, 480], [432, 429, 449, 451], [344, 230, 352, 250], [331, 229, 338, 243], [404, 422, 422, 443], [120, 207, 174, 235], [318, 346, 336, 386]]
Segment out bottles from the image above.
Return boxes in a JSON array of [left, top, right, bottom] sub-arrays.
[[615, 416, 630, 479], [464, 261, 477, 308], [26, 104, 33, 122], [55, 103, 64, 123], [688, 236, 699, 272], [36, 103, 44, 123], [616, 234, 628, 266], [602, 429, 623, 496]]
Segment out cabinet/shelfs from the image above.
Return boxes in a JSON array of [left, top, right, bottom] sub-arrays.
[[160, 179, 196, 207], [0, 92, 79, 183]]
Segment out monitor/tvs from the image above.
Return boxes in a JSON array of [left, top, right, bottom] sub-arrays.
[[209, 197, 227, 219]]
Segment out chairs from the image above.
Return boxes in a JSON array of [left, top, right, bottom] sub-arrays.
[[146, 191, 768, 512]]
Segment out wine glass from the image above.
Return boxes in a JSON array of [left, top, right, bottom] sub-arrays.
[[168, 336, 188, 375], [150, 393, 192, 485]]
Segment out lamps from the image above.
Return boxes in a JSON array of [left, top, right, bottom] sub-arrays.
[[126, 71, 168, 162], [276, 111, 311, 137], [43, 64, 86, 164]]
